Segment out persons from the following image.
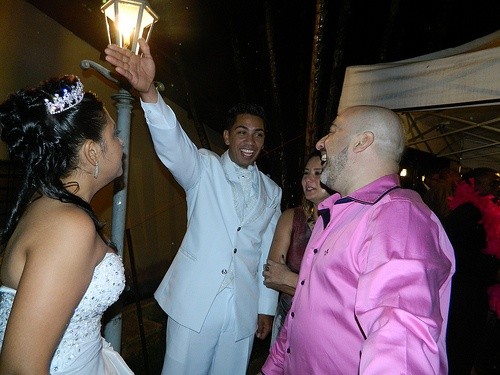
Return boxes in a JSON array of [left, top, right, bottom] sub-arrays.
[[105, 38, 282, 375], [263, 152, 335, 346], [258, 106, 456, 375], [0, 74, 129, 375], [402, 150, 500, 375]]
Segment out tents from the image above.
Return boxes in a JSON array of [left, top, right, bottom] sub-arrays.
[[337, 30, 499, 167]]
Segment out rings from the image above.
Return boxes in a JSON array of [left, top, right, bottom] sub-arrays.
[[264, 264, 269, 270]]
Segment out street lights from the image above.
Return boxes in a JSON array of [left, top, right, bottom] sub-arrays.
[[80, 0, 165, 355]]
